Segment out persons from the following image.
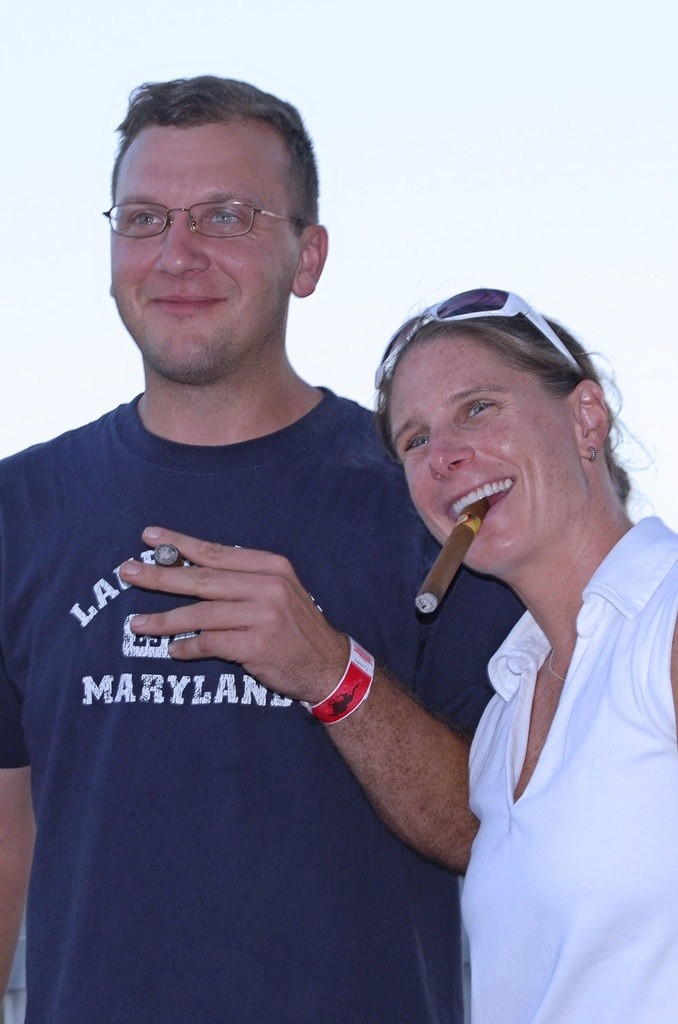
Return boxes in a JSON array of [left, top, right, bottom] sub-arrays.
[[378, 290, 678, 1024], [2, 72, 530, 1023]]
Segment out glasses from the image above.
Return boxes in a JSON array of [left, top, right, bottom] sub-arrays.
[[103, 200, 309, 239], [376, 289, 582, 389]]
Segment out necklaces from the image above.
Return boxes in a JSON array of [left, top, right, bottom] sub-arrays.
[[547, 648, 567, 682]]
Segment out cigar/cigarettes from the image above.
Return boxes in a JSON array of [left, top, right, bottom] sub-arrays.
[[154, 544, 182, 567], [414, 498, 490, 614]]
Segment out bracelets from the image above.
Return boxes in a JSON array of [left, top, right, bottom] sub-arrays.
[[300, 631, 376, 726]]
